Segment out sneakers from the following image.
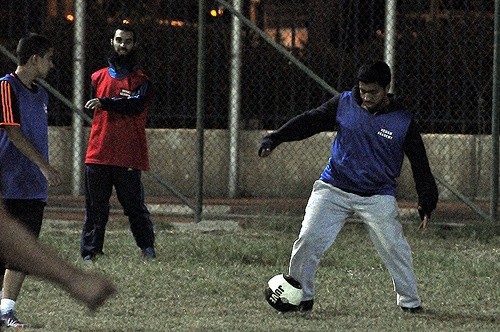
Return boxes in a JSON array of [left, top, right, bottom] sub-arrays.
[[0, 309, 28, 328]]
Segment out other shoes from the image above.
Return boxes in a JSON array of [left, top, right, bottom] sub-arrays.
[[141, 246, 157, 258], [300, 299, 314, 311], [401, 305, 422, 313]]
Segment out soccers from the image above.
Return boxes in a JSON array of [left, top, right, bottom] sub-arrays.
[[264, 273, 304, 312]]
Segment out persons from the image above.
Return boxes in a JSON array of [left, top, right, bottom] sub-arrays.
[[259, 61, 439, 315], [80, 23, 157, 261], [0, 32, 64, 329], [0, 203, 115, 312]]
